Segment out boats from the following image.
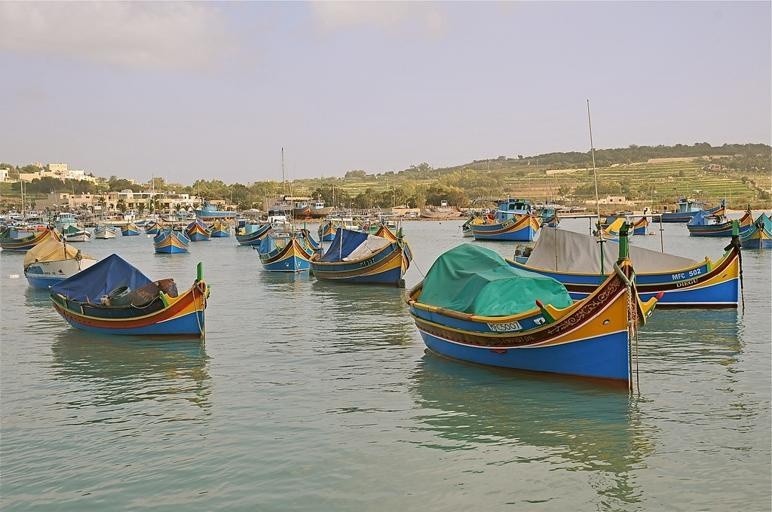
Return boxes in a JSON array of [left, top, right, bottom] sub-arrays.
[[256, 215, 414, 285], [403, 221, 659, 381], [461, 206, 560, 242], [599, 202, 772, 248], [24, 231, 98, 289], [0, 203, 274, 254], [47, 253, 208, 336], [504, 220, 744, 310]]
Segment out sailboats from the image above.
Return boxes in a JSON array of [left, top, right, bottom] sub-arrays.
[[268, 145, 334, 218]]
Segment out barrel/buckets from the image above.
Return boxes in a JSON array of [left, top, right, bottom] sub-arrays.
[[108, 284, 132, 299]]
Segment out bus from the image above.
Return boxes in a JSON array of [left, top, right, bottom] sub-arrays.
[[441, 200, 448, 208]]
